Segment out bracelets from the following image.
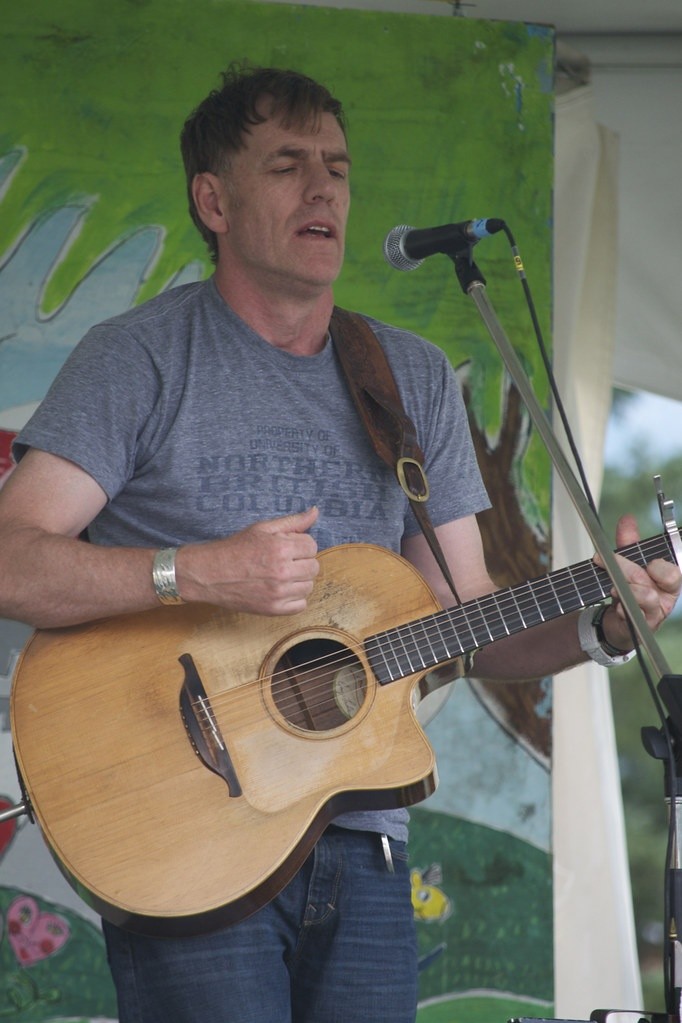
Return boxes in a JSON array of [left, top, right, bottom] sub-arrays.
[[593, 601, 631, 656], [152, 546, 185, 607]]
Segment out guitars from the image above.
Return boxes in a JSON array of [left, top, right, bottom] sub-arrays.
[[10, 527, 682, 941]]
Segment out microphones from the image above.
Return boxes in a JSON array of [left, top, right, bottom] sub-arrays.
[[382, 219, 491, 272]]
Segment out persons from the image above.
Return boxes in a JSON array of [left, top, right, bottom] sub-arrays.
[[0, 63, 682, 1022]]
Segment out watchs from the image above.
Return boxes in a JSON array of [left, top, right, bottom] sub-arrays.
[[576, 605, 639, 670]]
